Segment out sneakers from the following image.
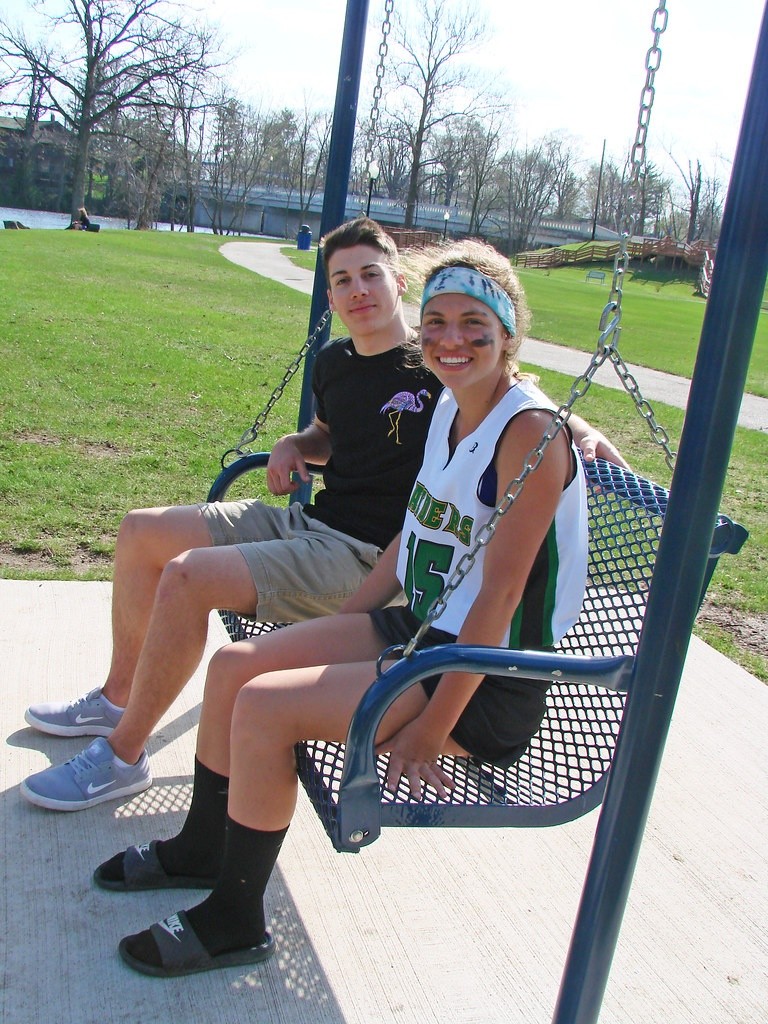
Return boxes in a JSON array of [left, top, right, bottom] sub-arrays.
[[21, 737, 152, 810], [25, 687, 127, 736]]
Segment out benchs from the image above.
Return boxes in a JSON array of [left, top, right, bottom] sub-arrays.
[[585, 270, 606, 284]]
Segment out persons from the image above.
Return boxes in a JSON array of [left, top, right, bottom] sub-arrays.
[[96, 237, 590, 978], [21, 217, 632, 815], [73, 207, 90, 231]]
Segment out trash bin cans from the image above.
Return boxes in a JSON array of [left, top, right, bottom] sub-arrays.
[[297, 224, 313, 250]]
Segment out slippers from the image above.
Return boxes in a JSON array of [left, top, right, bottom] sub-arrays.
[[93, 840, 220, 891], [119, 910, 275, 978]]
